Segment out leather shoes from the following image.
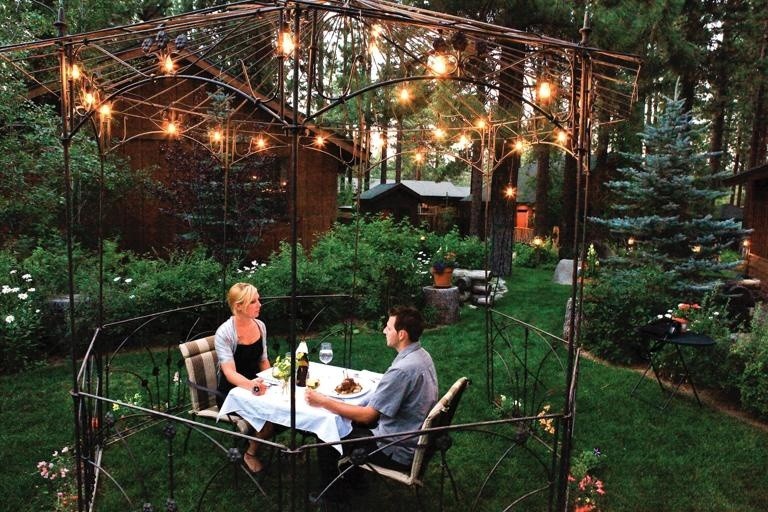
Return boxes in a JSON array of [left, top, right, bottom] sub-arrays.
[[241, 453, 270, 480]]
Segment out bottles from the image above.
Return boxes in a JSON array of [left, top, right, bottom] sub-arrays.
[[294, 338, 309, 386]]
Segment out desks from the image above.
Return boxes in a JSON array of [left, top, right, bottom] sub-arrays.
[[224, 359, 385, 511], [628, 327, 716, 411]]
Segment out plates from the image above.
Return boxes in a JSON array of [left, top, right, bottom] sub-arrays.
[[326, 377, 371, 398], [256, 367, 282, 383]]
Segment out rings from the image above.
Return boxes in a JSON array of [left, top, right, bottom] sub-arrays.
[[252, 384, 259, 394]]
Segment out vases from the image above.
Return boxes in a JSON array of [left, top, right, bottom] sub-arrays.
[[432, 267, 453, 289], [281, 378, 289, 395]]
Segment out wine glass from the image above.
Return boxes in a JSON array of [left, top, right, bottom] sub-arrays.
[[273, 362, 284, 397], [318, 342, 334, 379]]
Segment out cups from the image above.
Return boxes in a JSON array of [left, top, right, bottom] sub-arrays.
[[284, 352, 291, 364]]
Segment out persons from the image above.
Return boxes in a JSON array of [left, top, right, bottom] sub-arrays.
[[213, 282, 278, 478], [303, 306, 440, 512]]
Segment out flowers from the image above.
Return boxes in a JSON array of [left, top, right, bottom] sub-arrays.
[[429, 245, 459, 277], [272, 351, 300, 383], [654, 301, 709, 334]]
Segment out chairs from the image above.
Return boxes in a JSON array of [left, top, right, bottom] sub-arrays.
[[306, 375, 468, 510], [179, 334, 255, 488]]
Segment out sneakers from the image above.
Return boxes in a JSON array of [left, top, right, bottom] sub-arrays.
[[308, 491, 348, 508]]
